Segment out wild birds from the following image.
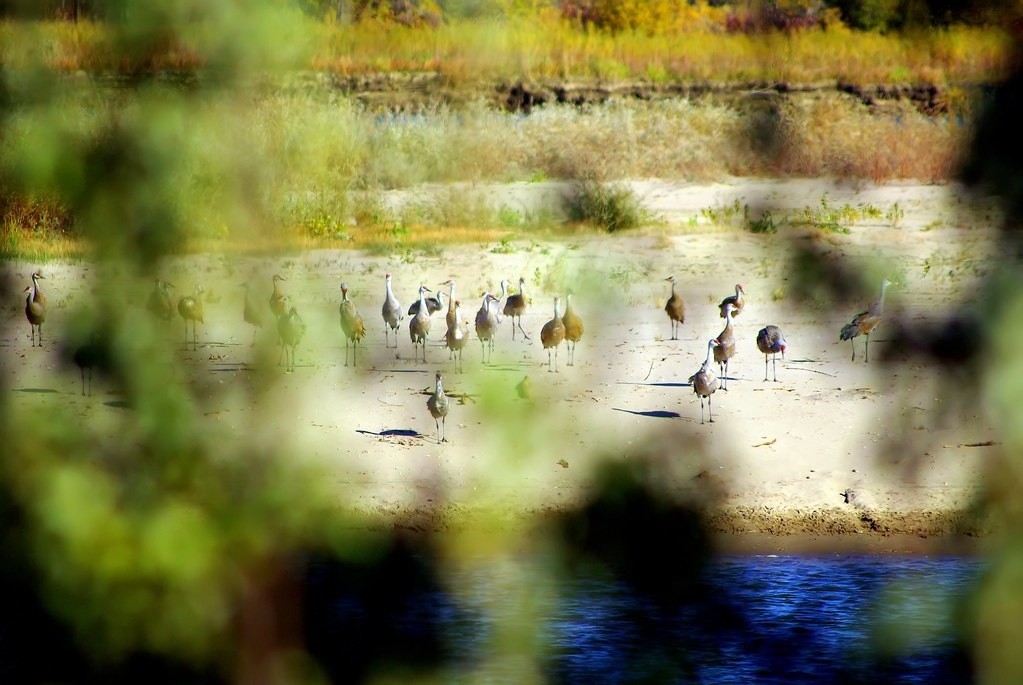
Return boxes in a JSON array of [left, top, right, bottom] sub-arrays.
[[756, 325, 787, 382], [426, 372, 450, 445], [269, 272, 307, 372], [408, 284, 449, 364], [713, 283, 747, 391], [438, 279, 470, 374], [178, 283, 206, 351], [238, 281, 268, 348], [475, 276, 530, 364], [21, 272, 47, 347], [339, 283, 366, 368], [146, 279, 176, 328], [663, 276, 685, 341], [71, 330, 103, 398], [381, 273, 404, 348], [540, 287, 585, 373], [839, 279, 892, 363], [687, 338, 724, 425]]
